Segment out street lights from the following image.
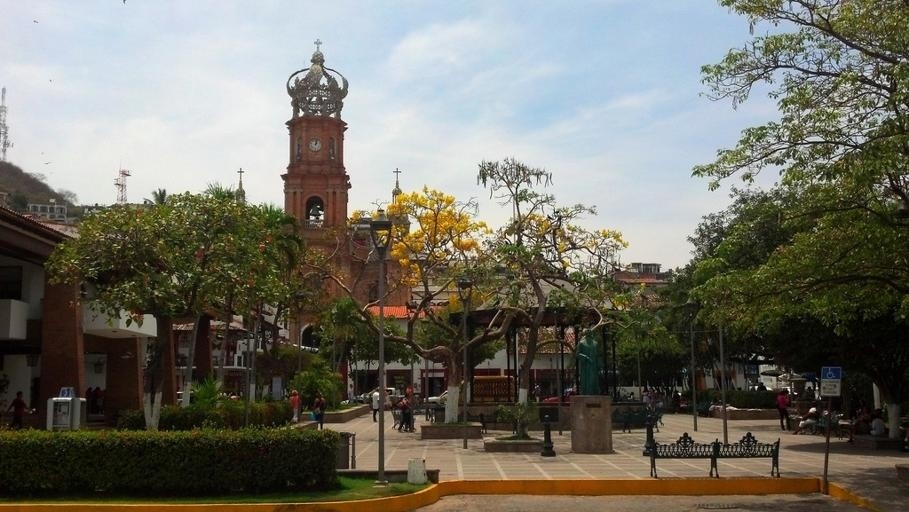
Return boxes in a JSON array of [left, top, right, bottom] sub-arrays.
[[369, 208, 394, 482], [457, 271, 475, 450]]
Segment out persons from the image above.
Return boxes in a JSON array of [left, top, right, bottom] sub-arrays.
[[86, 387, 106, 415], [775, 385, 886, 441], [6, 391, 29, 428], [575, 328, 602, 395], [289, 382, 416, 433], [642, 386, 681, 415]]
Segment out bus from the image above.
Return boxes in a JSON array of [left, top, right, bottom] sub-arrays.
[[569, 368, 689, 405]]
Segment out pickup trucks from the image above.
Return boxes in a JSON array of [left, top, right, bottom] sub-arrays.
[[362, 386, 405, 404]]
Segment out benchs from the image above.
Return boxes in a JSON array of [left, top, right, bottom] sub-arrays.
[[644, 432, 780, 478]]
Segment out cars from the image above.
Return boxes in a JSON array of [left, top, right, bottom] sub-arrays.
[[543, 390, 577, 405], [423, 391, 448, 405]]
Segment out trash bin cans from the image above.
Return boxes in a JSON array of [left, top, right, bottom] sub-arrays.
[[569, 393, 614, 456]]
